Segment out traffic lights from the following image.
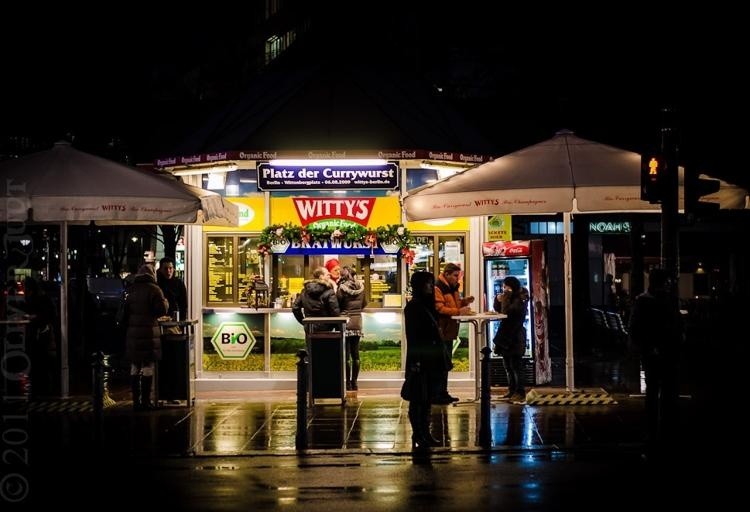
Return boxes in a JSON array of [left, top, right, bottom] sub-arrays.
[[686, 137, 721, 221], [639, 139, 670, 205]]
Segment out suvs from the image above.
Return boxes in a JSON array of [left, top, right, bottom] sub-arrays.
[[71, 276, 126, 317]]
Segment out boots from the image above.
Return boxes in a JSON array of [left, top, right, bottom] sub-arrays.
[[346, 359, 360, 391], [129, 374, 160, 411], [408, 407, 441, 448]]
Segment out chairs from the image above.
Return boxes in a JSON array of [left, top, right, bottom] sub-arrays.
[[590, 307, 628, 336]]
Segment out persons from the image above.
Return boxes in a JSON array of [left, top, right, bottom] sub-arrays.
[[433, 263, 474, 404], [325, 258, 342, 284], [157, 258, 188, 335], [400, 271, 452, 447], [337, 265, 368, 391], [293, 266, 341, 359], [629, 269, 680, 440], [492, 276, 528, 405], [120, 264, 171, 408]]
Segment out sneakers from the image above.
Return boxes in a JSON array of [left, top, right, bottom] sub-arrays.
[[504, 391, 526, 399], [433, 394, 459, 404]]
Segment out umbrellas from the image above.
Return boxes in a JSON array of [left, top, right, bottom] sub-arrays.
[[0, 138, 239, 371], [404, 127, 750, 394]]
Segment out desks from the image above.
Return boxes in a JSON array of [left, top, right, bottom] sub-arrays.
[[448, 309, 506, 411]]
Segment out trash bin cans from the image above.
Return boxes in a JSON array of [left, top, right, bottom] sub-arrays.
[[302, 316, 350, 407], [156, 320, 199, 407]]
